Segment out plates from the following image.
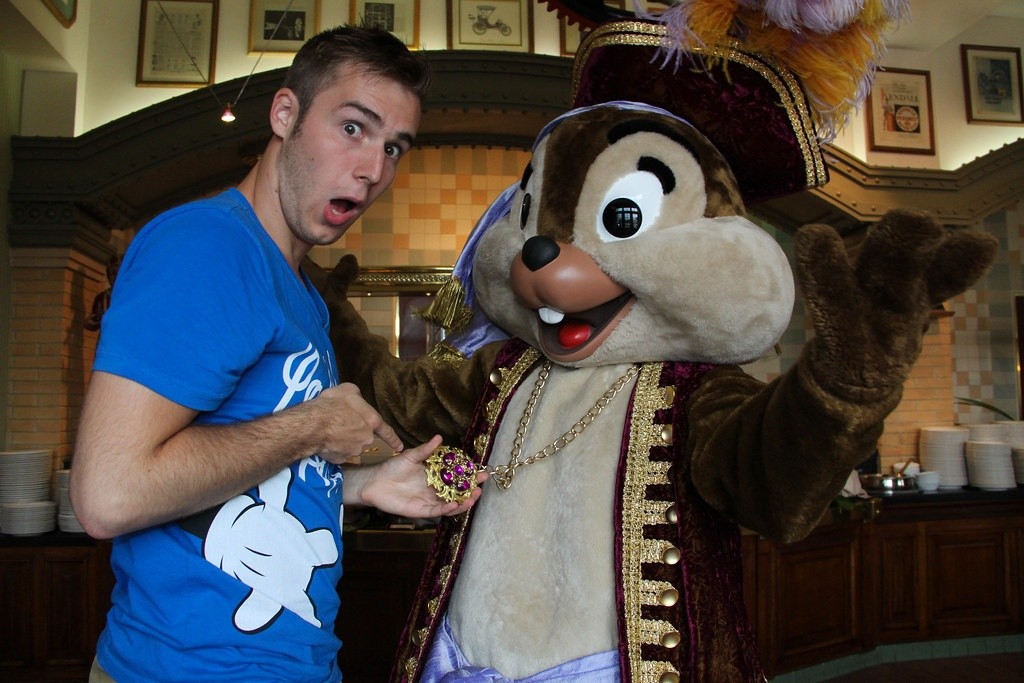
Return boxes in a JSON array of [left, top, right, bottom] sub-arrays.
[[0, 448, 86, 534], [919, 419, 1024, 491]]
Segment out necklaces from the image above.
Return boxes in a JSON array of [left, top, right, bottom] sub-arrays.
[[424, 358, 642, 506]]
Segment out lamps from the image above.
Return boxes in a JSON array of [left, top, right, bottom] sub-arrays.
[[222, 103, 236, 123]]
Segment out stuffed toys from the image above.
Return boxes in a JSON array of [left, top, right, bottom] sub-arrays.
[[304, 0, 1001, 683]]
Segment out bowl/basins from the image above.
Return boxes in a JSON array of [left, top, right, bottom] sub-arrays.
[[915, 472, 940, 491], [893, 461, 920, 477], [867, 473, 915, 490]]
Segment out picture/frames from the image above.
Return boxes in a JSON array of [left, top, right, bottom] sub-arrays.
[[863, 66, 936, 156], [557, 0, 625, 55], [446, 0, 535, 53], [247, 0, 321, 56], [349, 0, 420, 49], [42, 0, 79, 28], [960, 43, 1024, 125]]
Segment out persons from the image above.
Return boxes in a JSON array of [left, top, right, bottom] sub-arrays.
[[67, 21, 488, 683]]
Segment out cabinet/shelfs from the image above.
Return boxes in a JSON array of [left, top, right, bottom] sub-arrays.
[[0, 544, 424, 683], [744, 499, 1024, 674]]
